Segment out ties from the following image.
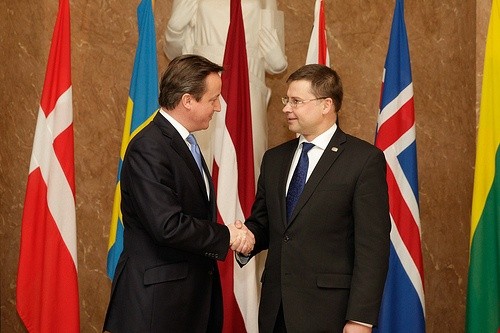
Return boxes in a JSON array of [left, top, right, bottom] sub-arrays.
[[186, 134, 203, 178], [286, 142, 316, 223]]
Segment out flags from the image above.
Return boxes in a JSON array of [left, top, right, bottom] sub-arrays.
[[374, 0, 427, 333], [304, 0, 330, 68], [465, 0, 500, 333], [16, 0, 80, 333], [211, 0, 258, 333], [106, 0, 161, 281]]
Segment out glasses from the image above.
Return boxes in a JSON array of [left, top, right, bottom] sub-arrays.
[[281, 96, 327, 109]]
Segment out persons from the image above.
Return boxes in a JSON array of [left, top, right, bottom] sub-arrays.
[[163, 0, 288, 163], [101, 54, 255, 333], [230, 64, 389, 333]]
